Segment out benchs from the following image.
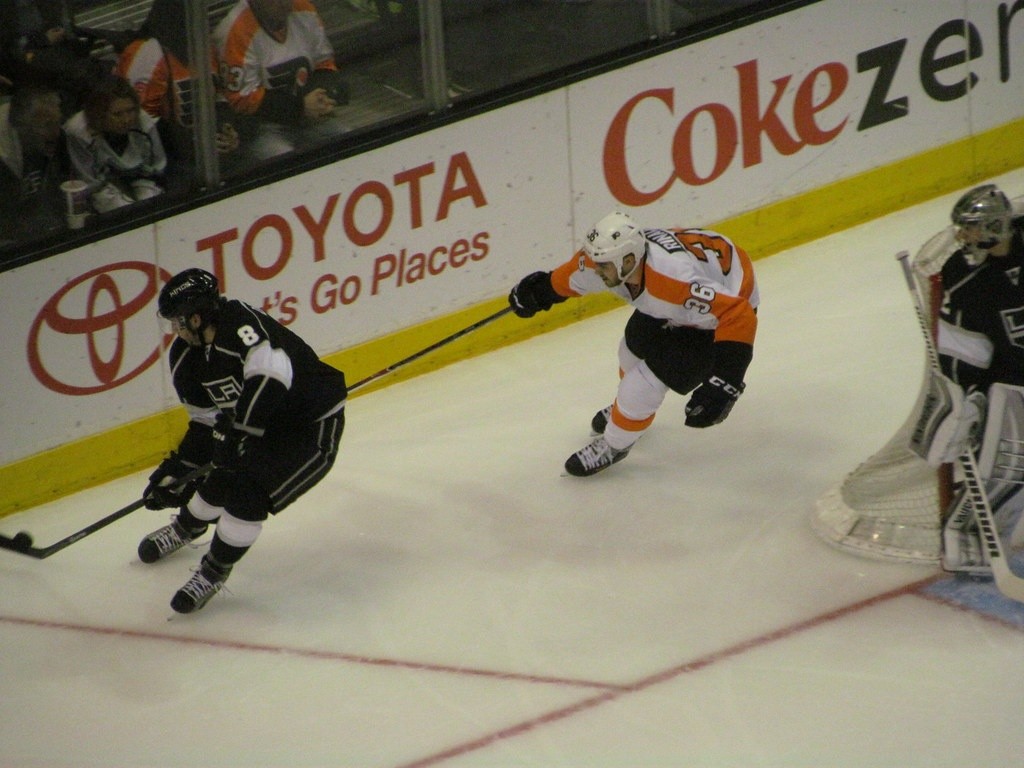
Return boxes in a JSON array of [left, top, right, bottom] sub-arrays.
[[74, 0, 472, 153]]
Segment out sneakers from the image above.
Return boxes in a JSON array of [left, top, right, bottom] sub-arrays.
[[166, 556, 232, 621], [591, 405, 617, 437], [561, 438, 634, 478], [136, 516, 209, 564]]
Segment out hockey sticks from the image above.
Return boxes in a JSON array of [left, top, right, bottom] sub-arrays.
[[895, 251, 1023, 603], [1, 458, 221, 562], [345, 307, 514, 396]]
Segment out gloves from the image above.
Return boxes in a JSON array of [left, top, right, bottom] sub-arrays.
[[508, 269, 570, 319], [143, 454, 207, 510], [684, 372, 747, 429], [211, 414, 258, 475]]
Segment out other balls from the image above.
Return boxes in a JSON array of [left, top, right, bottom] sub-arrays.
[[15, 531, 33, 548]]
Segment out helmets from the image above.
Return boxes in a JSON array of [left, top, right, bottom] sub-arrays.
[[583, 212, 646, 268], [157, 267, 217, 321], [953, 183, 1014, 267]]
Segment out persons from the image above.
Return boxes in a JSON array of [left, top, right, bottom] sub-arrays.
[[63, 74, 166, 213], [508, 210, 759, 478], [139, 268, 346, 620], [0, 0, 347, 224], [913, 184, 1024, 578]]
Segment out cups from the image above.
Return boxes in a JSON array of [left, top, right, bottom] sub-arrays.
[[60, 180, 87, 229]]
